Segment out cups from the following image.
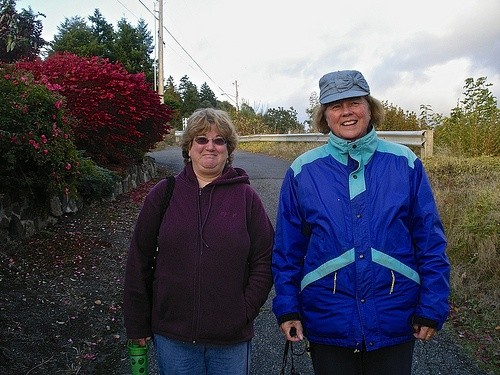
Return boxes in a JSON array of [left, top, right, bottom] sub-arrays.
[[127, 340, 148, 375]]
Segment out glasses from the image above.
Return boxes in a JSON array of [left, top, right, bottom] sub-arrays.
[[194, 136, 227, 146]]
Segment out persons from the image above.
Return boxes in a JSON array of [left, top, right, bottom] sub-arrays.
[[124, 108, 275, 375], [270, 69, 450, 374]]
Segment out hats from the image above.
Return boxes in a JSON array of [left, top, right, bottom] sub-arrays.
[[319, 70, 370, 105]]
